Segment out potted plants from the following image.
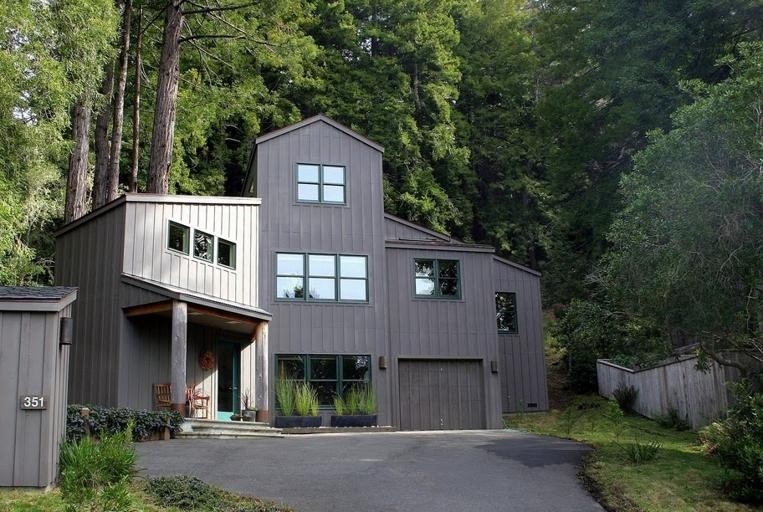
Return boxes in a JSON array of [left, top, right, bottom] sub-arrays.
[[237, 387, 256, 422], [327, 378, 378, 427], [273, 377, 322, 427]]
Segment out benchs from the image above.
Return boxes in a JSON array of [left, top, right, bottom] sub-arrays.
[[152, 383, 210, 420]]
